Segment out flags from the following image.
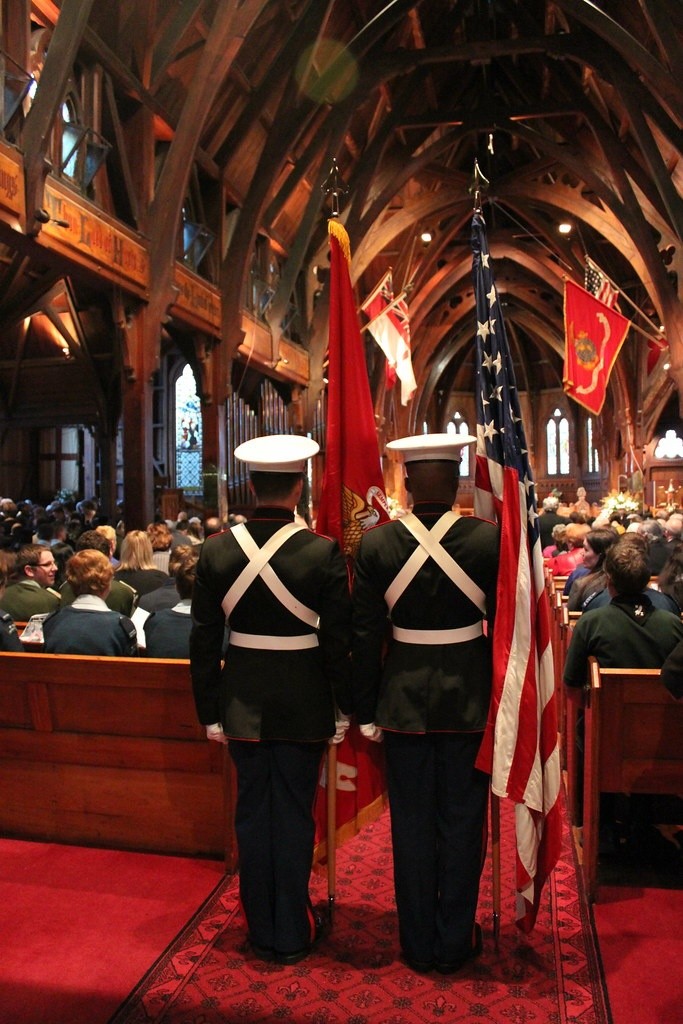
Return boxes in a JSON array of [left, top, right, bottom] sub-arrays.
[[585, 260, 619, 308], [317, 217, 388, 571], [470, 216, 561, 938], [368, 293, 417, 406], [361, 270, 396, 388], [561, 278, 630, 417]]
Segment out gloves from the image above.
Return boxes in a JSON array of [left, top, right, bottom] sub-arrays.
[[206, 723, 228, 744], [359, 722, 384, 743], [327, 709, 351, 744]]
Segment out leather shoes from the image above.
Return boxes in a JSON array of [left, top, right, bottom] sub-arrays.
[[273, 909, 329, 964], [405, 953, 437, 972], [435, 922, 482, 975], [245, 929, 272, 959]]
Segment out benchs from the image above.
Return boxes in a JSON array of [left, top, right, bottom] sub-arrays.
[[543, 558, 683, 906], [1, 620, 240, 876]]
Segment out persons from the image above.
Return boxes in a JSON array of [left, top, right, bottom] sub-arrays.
[[536, 497, 683, 860], [189, 435, 354, 963], [0, 495, 249, 657], [352, 433, 499, 973]]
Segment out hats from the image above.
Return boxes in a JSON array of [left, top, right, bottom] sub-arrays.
[[386, 433, 479, 462], [235, 435, 320, 473]]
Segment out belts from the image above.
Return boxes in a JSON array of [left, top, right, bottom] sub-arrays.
[[390, 618, 484, 645], [228, 631, 320, 650]]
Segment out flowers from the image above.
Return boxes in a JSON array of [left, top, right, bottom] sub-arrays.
[[598, 488, 641, 515]]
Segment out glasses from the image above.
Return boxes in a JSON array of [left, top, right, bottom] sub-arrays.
[[26, 561, 52, 567]]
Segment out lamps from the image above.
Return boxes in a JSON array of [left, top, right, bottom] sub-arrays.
[[421, 229, 435, 247], [34, 208, 70, 229]]
